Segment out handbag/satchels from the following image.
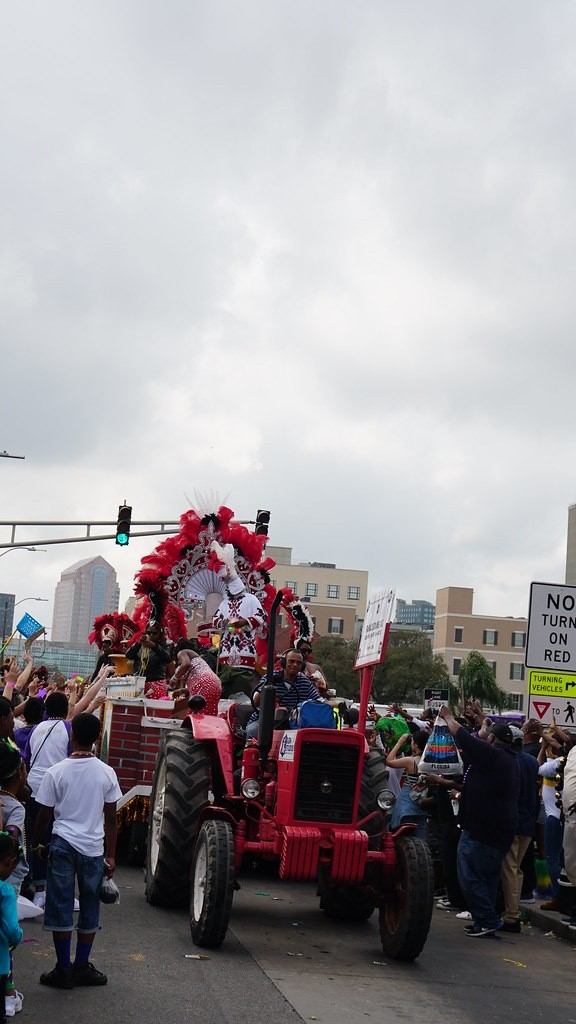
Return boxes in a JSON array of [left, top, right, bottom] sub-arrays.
[[375, 713, 413, 755], [100, 858, 120, 906], [418, 716, 464, 776]]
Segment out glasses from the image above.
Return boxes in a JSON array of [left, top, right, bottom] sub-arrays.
[[299, 648, 313, 654], [146, 631, 158, 635]]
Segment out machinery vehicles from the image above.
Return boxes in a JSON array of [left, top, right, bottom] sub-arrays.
[[98, 589, 437, 963]]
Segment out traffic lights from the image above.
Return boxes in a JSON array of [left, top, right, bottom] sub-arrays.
[[254, 509, 271, 550], [113, 504, 133, 547]]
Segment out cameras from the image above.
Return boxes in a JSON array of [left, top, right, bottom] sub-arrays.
[[406, 734, 413, 742]]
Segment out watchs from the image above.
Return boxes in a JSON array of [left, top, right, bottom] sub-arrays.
[[455, 792, 461, 799]]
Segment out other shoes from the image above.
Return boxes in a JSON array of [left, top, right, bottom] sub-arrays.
[[519, 896, 535, 902], [433, 888, 448, 899], [540, 900, 561, 911], [568, 922, 576, 929], [561, 916, 576, 925]]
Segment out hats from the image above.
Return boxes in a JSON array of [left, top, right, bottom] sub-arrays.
[[490, 725, 513, 743]]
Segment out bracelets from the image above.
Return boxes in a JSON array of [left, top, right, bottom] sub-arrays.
[[474, 714, 478, 718], [77, 694, 80, 698], [6, 682, 14, 687]]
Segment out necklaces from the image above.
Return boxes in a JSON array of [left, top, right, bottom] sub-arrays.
[[48, 717, 63, 720], [1, 789, 27, 860]]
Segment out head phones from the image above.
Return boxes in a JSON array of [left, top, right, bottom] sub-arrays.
[[281, 648, 306, 672]]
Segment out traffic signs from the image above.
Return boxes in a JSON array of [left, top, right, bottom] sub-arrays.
[[526, 668, 576, 729]]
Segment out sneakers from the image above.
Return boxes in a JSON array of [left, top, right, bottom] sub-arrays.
[[457, 910, 473, 920], [71, 957, 107, 987], [464, 920, 504, 936], [40, 963, 74, 989], [437, 902, 462, 909], [438, 897, 452, 903], [4, 989, 24, 1017], [33, 891, 46, 907], [73, 897, 81, 911]]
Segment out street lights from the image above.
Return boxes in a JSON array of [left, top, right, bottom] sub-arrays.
[[1, 598, 49, 677], [16, 626, 52, 665]]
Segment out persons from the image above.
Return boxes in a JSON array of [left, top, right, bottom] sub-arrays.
[[337, 697, 576, 938], [243, 638, 342, 745], [169, 637, 221, 716], [212, 577, 265, 676], [0, 650, 120, 1024], [89, 619, 217, 702]]
